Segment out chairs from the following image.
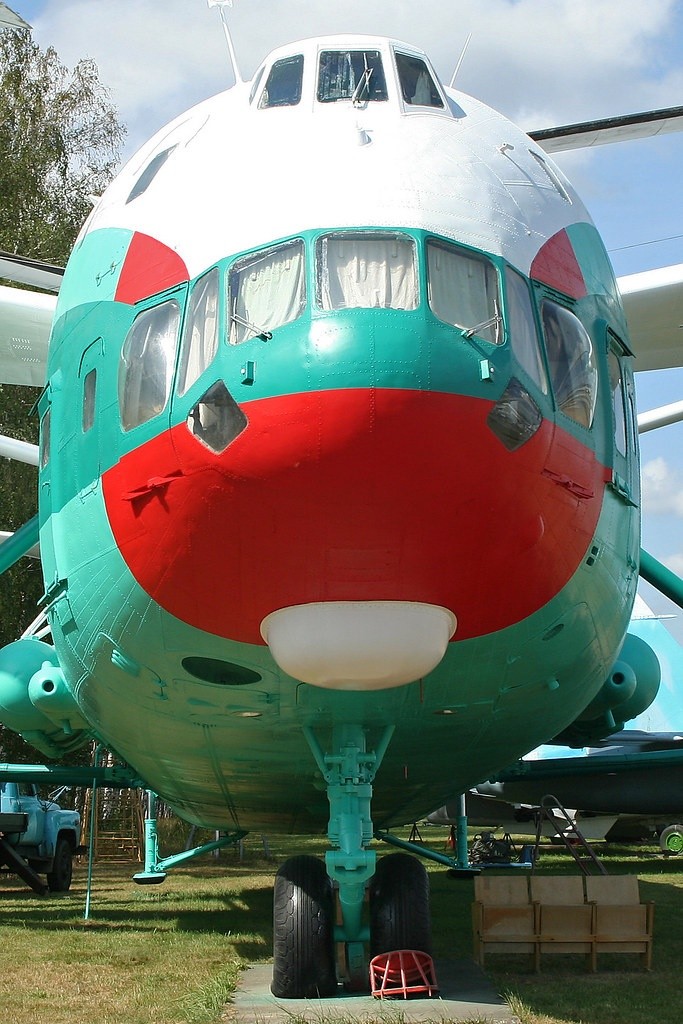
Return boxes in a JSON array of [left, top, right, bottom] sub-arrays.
[[470, 874, 655, 973]]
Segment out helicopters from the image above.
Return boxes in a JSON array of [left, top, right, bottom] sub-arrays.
[[0, 0, 681, 1007]]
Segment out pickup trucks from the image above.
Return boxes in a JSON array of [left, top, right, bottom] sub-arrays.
[[0, 780, 87, 895]]
